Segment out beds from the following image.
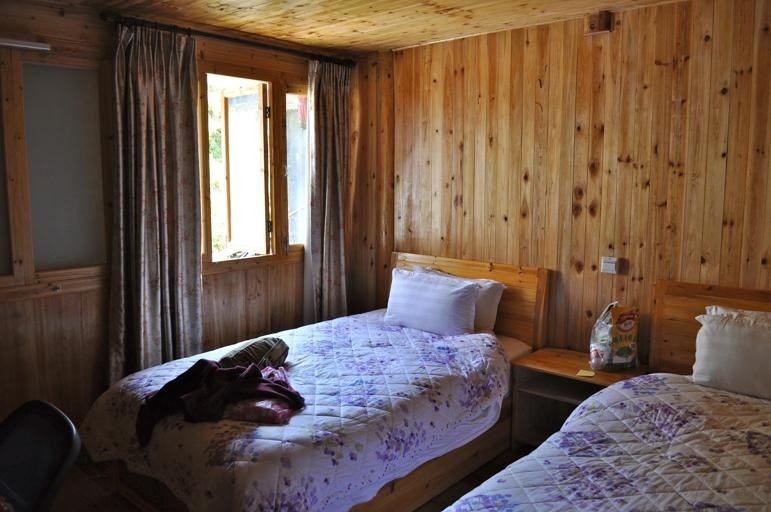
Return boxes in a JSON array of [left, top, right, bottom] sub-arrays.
[[78, 252, 552, 512], [440, 279, 771, 512]]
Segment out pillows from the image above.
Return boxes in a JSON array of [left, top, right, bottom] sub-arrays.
[[414, 265, 507, 333], [385, 268, 481, 341], [692, 316, 771, 400], [704, 305, 771, 318]]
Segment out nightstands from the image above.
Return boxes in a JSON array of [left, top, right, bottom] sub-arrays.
[[510, 347, 650, 452]]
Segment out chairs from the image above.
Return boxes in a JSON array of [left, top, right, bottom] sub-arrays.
[[0, 398, 81, 512]]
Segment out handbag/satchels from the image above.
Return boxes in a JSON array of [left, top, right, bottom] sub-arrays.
[[220, 335, 289, 369]]
